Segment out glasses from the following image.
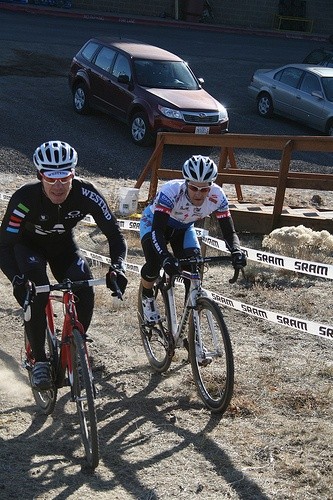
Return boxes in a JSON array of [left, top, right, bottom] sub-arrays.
[[42, 177, 73, 185], [187, 183, 211, 193]]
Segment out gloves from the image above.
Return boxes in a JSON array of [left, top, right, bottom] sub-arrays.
[[104, 266, 127, 298], [162, 256, 181, 275], [12, 278, 37, 310], [231, 249, 248, 269]]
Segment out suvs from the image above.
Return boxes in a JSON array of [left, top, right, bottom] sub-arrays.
[[68, 36, 229, 147]]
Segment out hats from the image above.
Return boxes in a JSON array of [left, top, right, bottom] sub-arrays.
[[40, 171, 73, 179]]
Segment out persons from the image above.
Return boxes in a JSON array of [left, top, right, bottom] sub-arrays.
[[0, 140, 128, 391], [139, 155, 247, 367]]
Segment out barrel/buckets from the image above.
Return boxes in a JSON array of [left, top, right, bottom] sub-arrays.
[[118, 187, 140, 216]]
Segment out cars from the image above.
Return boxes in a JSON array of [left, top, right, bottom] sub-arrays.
[[247, 62, 333, 136]]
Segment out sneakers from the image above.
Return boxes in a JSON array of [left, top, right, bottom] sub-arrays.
[[31, 363, 50, 390], [73, 340, 89, 380], [142, 297, 161, 322], [195, 340, 210, 364]]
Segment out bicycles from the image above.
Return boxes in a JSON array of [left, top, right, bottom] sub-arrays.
[[137, 250, 248, 411], [20, 270, 123, 469]]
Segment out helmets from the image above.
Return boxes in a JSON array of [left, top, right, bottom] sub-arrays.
[[32, 140, 78, 171], [181, 156, 218, 183]]
